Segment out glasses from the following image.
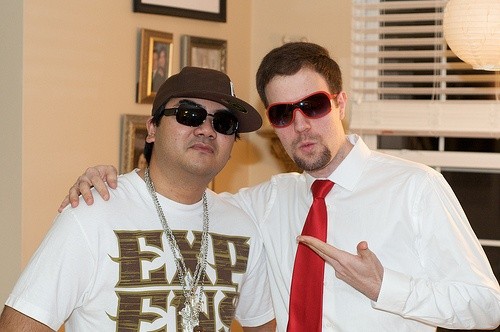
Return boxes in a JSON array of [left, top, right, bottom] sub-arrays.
[[266, 91, 339, 127], [161, 103, 240, 135]]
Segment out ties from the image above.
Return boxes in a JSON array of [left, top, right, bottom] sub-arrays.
[[287, 180, 334, 332]]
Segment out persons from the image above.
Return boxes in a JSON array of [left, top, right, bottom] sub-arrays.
[[59, 42, 500, 332], [0, 66, 277, 332]]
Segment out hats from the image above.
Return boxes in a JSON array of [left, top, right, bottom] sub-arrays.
[[152, 67, 262, 133]]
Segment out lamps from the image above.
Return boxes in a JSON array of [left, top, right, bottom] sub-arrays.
[[442, 0, 500, 72]]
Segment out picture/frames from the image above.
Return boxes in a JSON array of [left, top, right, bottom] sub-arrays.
[[138, 28, 173, 104], [119, 115, 150, 174], [133, 0, 227, 23], [180, 35, 227, 73]]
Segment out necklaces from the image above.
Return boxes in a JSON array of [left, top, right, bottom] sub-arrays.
[[145, 165, 208, 332]]
